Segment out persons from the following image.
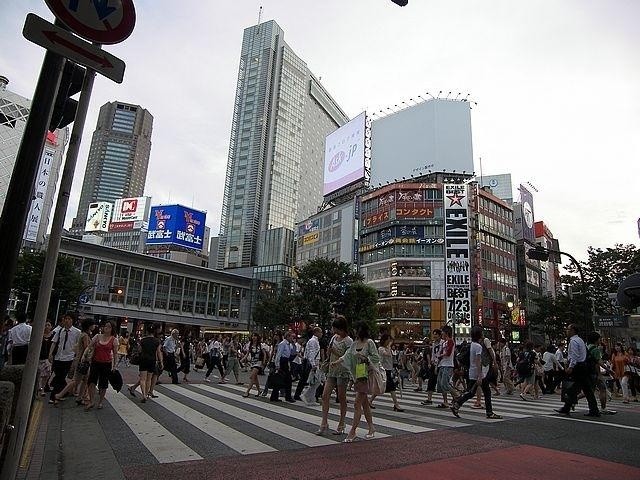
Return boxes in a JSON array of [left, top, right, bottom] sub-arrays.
[[136, 324, 164, 402], [0, 310, 34, 364], [553, 324, 603, 417], [368, 325, 568, 419], [597, 342, 640, 403], [36, 310, 143, 410], [240, 327, 323, 405], [128, 323, 162, 397], [314, 317, 380, 443], [156, 328, 250, 385], [571, 332, 618, 415]]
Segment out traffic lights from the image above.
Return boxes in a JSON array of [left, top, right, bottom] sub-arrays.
[[109, 288, 124, 295], [507, 295, 514, 311], [51, 60, 97, 135]]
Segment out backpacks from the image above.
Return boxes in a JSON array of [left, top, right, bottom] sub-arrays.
[[517, 351, 534, 378]]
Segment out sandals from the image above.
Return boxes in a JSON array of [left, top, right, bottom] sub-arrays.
[[486, 413, 503, 419], [41, 384, 103, 412], [421, 399, 461, 418]]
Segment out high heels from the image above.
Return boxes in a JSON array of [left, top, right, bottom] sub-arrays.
[[394, 405, 404, 412], [128, 386, 159, 403], [368, 400, 375, 409], [315, 424, 376, 443]]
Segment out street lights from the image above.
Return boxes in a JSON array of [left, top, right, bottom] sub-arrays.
[[525, 245, 588, 345], [391, 0, 409, 8], [453, 286, 482, 345]]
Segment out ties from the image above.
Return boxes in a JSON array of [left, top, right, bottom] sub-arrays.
[[63, 330, 69, 350]]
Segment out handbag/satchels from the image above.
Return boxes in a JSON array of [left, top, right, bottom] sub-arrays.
[[82, 334, 99, 361], [354, 363, 386, 395]]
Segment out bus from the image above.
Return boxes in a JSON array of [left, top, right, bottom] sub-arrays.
[[198, 330, 250, 346]]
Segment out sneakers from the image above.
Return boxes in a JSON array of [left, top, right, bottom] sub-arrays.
[[492, 390, 543, 400], [203, 375, 244, 384], [554, 404, 617, 416]]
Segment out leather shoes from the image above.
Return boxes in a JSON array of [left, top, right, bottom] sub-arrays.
[[168, 371, 192, 384], [242, 390, 301, 402]]
